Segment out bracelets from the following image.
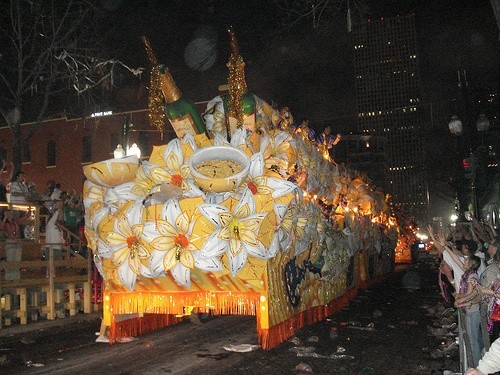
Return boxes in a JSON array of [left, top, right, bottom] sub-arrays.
[[442, 244, 446, 247]]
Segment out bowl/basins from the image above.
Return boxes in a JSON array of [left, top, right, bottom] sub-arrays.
[[188, 146, 250, 190], [81, 154, 138, 191]]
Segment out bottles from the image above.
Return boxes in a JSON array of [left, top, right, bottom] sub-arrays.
[[156, 63, 210, 142], [224, 52, 257, 146]]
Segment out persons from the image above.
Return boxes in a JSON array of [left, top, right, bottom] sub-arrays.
[[426, 211, 500, 368], [279, 105, 296, 131], [296, 118, 315, 141], [464, 338, 500, 375], [317, 123, 341, 149], [0, 170, 84, 249]]
